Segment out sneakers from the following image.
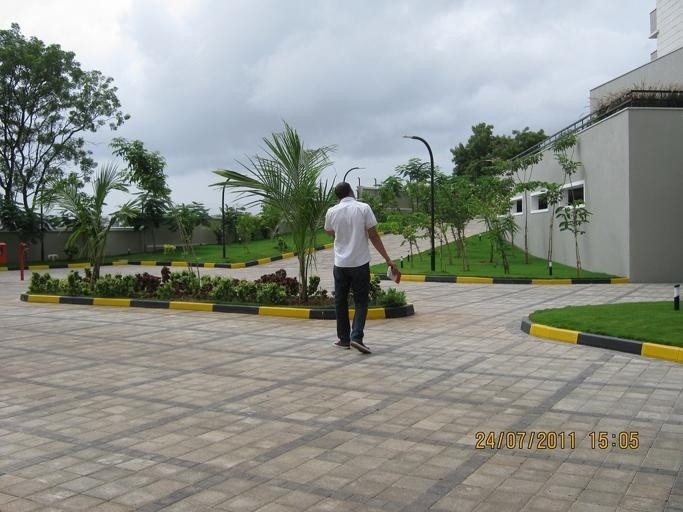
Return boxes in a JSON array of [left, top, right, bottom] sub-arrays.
[[332, 337, 372, 354]]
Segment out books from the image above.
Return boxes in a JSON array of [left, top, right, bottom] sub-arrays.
[[386, 265, 401, 284]]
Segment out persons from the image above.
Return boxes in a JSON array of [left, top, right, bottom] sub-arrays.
[[322, 181, 398, 354]]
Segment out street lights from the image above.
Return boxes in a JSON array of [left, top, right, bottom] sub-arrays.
[[402, 134, 435, 272]]
